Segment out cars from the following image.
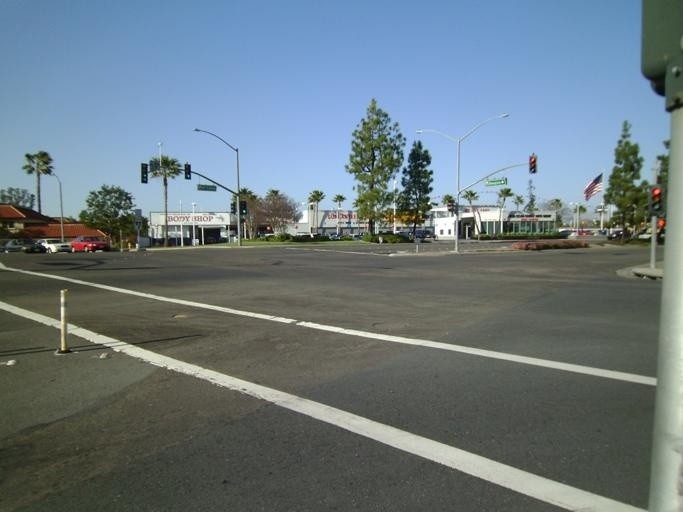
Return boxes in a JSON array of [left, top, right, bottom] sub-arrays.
[[195, 232, 366, 244], [68, 235, 108, 253], [375, 227, 437, 238], [0, 238, 46, 254], [559, 225, 666, 241], [36, 238, 72, 253]]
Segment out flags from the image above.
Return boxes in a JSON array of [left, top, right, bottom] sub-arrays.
[[584, 171, 605, 201]]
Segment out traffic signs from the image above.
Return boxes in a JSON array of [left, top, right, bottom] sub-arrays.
[[198, 184, 216, 191], [485, 178, 507, 186]]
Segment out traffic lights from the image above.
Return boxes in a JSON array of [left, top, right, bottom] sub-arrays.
[[185, 164, 191, 179], [649, 185, 664, 214], [529, 154, 537, 173], [141, 164, 147, 183], [230, 202, 235, 215], [240, 200, 247, 215]]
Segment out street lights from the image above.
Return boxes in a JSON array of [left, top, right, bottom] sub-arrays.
[[569, 200, 589, 237], [393, 180, 398, 234], [415, 112, 510, 253], [192, 128, 242, 248], [190, 201, 197, 246], [597, 202, 608, 229], [51, 173, 64, 243]]
